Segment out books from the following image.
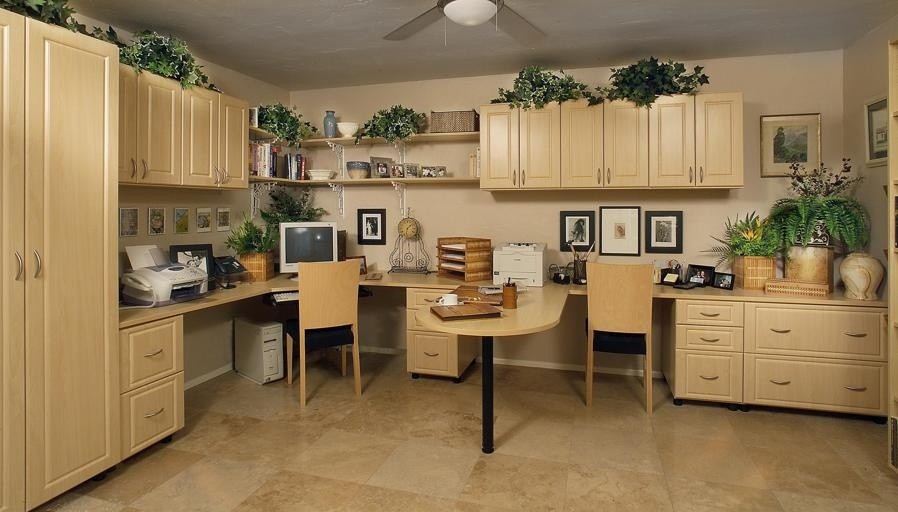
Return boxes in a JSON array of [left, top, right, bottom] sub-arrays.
[[248, 138, 308, 180]]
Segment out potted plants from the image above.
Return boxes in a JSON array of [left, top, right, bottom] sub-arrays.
[[223, 211, 280, 282]]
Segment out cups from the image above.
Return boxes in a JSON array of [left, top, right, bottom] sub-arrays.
[[572, 260, 587, 285], [501, 285, 518, 309], [552, 272, 569, 284], [439, 293, 457, 306]]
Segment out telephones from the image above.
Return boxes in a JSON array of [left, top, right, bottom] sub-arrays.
[[121, 265, 208, 307], [214, 255, 245, 275]]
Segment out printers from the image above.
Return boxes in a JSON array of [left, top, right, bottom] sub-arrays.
[[120, 248, 209, 307]]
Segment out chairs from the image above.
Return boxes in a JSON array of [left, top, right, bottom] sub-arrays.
[[286, 259, 362, 408], [584, 261, 655, 410]]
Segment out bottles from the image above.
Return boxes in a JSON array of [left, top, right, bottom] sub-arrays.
[[467, 151, 476, 177]]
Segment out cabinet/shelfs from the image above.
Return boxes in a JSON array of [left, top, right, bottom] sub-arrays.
[[561, 98, 648, 189], [117, 62, 183, 188], [182, 82, 251, 188], [250, 123, 478, 184], [648, 92, 745, 189], [745, 297, 887, 415], [479, 100, 559, 188], [0, 9, 123, 511], [884, 38, 897, 474], [436, 236, 493, 282], [660, 296, 747, 405]]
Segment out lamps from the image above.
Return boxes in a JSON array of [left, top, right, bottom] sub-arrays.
[[442, 0, 497, 28]]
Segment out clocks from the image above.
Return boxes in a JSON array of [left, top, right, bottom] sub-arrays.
[[386, 207, 432, 277]]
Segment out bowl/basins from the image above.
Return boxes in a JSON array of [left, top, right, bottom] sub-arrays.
[[336, 122, 359, 138], [305, 169, 335, 181], [346, 161, 370, 179]]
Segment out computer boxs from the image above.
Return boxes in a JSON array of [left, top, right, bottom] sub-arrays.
[[233, 315, 284, 386]]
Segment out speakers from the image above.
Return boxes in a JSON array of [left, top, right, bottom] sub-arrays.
[[337, 230, 346, 262]]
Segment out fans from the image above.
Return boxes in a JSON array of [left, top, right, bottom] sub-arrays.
[[381, 0, 549, 43]]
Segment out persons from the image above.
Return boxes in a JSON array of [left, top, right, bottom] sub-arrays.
[[774, 125, 790, 157], [701, 271, 706, 280], [719, 278, 729, 286], [693, 271, 701, 277], [185, 255, 206, 270]]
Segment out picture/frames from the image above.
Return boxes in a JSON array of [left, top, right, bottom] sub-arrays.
[[367, 155, 448, 179], [560, 210, 594, 251], [645, 209, 685, 253], [215, 207, 231, 232], [195, 207, 213, 234], [685, 264, 715, 286], [599, 206, 641, 255], [148, 207, 167, 236], [864, 93, 888, 167], [169, 243, 216, 290], [119, 207, 139, 238], [758, 111, 822, 180], [710, 272, 735, 290], [356, 207, 387, 245], [173, 207, 190, 235]]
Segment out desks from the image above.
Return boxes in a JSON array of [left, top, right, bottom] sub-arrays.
[[357, 260, 673, 384], [414, 287, 567, 453], [117, 264, 301, 457]]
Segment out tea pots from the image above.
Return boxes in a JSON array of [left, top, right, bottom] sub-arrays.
[[546, 263, 561, 281]]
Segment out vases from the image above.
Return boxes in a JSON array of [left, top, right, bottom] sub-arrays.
[[794, 218, 832, 246], [730, 253, 776, 291], [323, 110, 336, 138], [838, 252, 885, 301]]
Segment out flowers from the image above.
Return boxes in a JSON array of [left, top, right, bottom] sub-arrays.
[[699, 209, 777, 272], [765, 155, 873, 253]]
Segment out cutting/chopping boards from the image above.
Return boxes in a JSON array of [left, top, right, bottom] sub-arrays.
[[432, 284, 504, 306]]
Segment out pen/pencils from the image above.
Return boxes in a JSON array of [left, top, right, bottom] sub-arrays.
[[567, 241, 596, 261], [508, 277, 510, 287]]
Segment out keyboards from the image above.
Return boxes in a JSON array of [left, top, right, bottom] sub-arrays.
[[273, 292, 299, 303]]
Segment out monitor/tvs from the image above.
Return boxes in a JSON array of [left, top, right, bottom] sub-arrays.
[[279, 222, 338, 281]]
